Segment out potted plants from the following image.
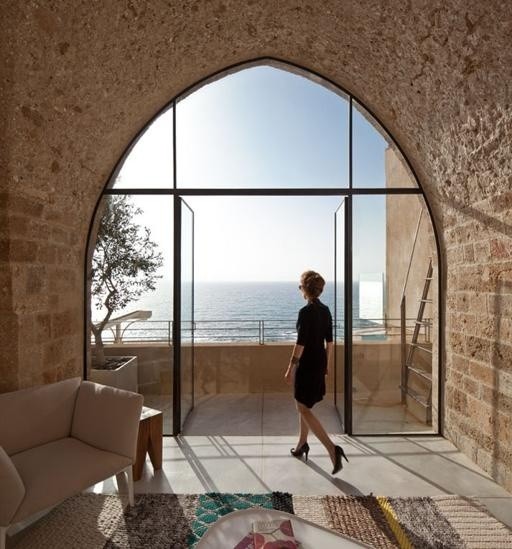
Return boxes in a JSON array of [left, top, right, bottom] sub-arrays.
[[86, 190, 164, 395]]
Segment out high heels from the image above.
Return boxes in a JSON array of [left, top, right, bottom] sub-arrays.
[[290, 442, 309, 461], [331, 446, 348, 474]]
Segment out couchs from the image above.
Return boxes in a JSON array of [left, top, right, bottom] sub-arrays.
[[1, 377, 145, 549]]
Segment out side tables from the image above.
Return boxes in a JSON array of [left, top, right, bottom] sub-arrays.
[[133, 406, 163, 481]]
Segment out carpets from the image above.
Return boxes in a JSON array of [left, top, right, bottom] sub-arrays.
[[5, 493, 512, 548]]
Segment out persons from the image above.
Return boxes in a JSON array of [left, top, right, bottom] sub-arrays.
[[283, 269, 350, 476]]
